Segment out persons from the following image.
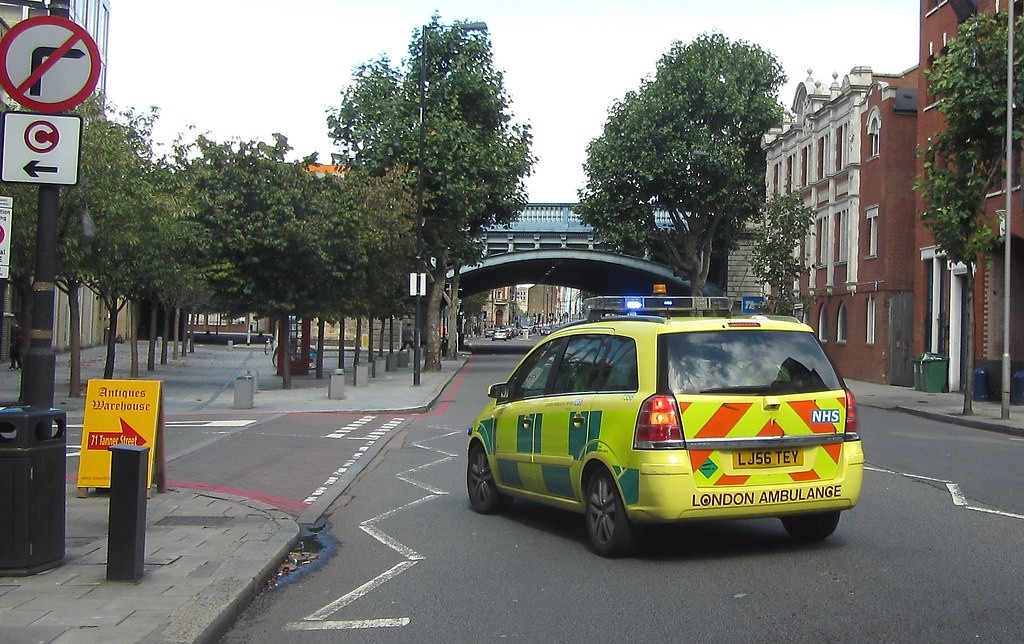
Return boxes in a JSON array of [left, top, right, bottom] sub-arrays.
[[398, 322, 414, 352], [8, 318, 21, 369]]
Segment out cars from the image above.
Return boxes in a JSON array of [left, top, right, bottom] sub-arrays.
[[467, 296, 865, 557], [485, 325, 519, 341], [520, 325, 532, 335], [537, 326, 551, 336]]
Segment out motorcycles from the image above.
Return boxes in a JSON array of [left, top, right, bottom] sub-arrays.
[[532, 327, 536, 334]]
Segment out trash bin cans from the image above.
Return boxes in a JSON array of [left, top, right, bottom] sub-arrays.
[[0, 403, 67, 577], [911, 350, 949, 392]]
